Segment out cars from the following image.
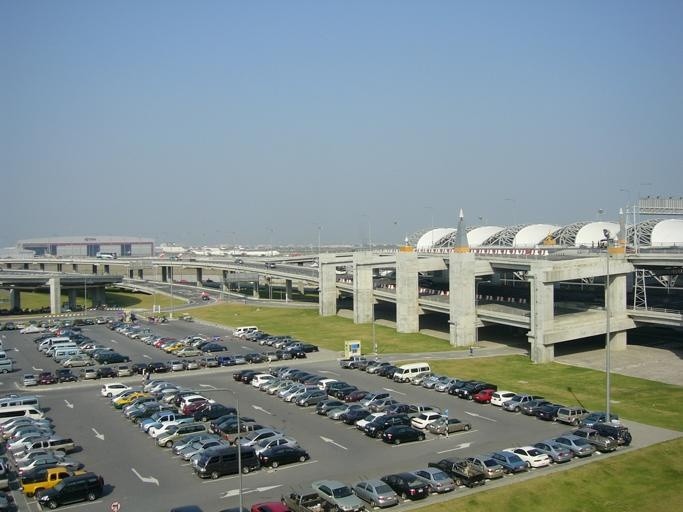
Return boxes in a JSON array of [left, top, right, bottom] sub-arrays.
[[536, 404, 565, 422], [101, 380, 309, 480], [486, 450, 528, 475], [201, 278, 220, 287], [465, 455, 504, 479], [490, 391, 519, 406], [381, 472, 429, 501], [553, 433, 595, 457], [178, 278, 188, 286], [502, 445, 550, 468], [284, 354, 476, 444], [531, 440, 572, 463], [522, 399, 552, 416], [312, 479, 365, 511], [1, 306, 318, 386], [502, 394, 535, 413], [250, 501, 290, 511], [264, 262, 274, 268], [234, 259, 243, 264], [474, 389, 496, 404], [354, 479, 398, 508], [1, 394, 106, 511], [200, 292, 209, 301], [582, 412, 617, 427], [414, 467, 455, 494]]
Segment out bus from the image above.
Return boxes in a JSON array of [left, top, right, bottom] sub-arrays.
[[96, 251, 116, 260]]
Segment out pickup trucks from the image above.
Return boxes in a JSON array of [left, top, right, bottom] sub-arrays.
[[281, 488, 335, 511], [427, 457, 484, 487], [569, 427, 617, 451]]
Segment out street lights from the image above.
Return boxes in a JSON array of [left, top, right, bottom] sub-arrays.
[[228, 182, 683, 258], [597, 229, 616, 423], [167, 239, 175, 314]]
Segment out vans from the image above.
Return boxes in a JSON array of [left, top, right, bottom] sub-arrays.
[[556, 407, 591, 426]]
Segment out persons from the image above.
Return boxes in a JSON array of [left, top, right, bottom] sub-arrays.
[[206, 428, 214, 434]]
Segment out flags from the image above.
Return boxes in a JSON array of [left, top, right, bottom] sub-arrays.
[[412, 247, 549, 257], [338, 276, 527, 304]]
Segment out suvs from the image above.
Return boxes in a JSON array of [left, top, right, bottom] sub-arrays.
[[590, 424, 631, 445], [458, 381, 498, 400]]
